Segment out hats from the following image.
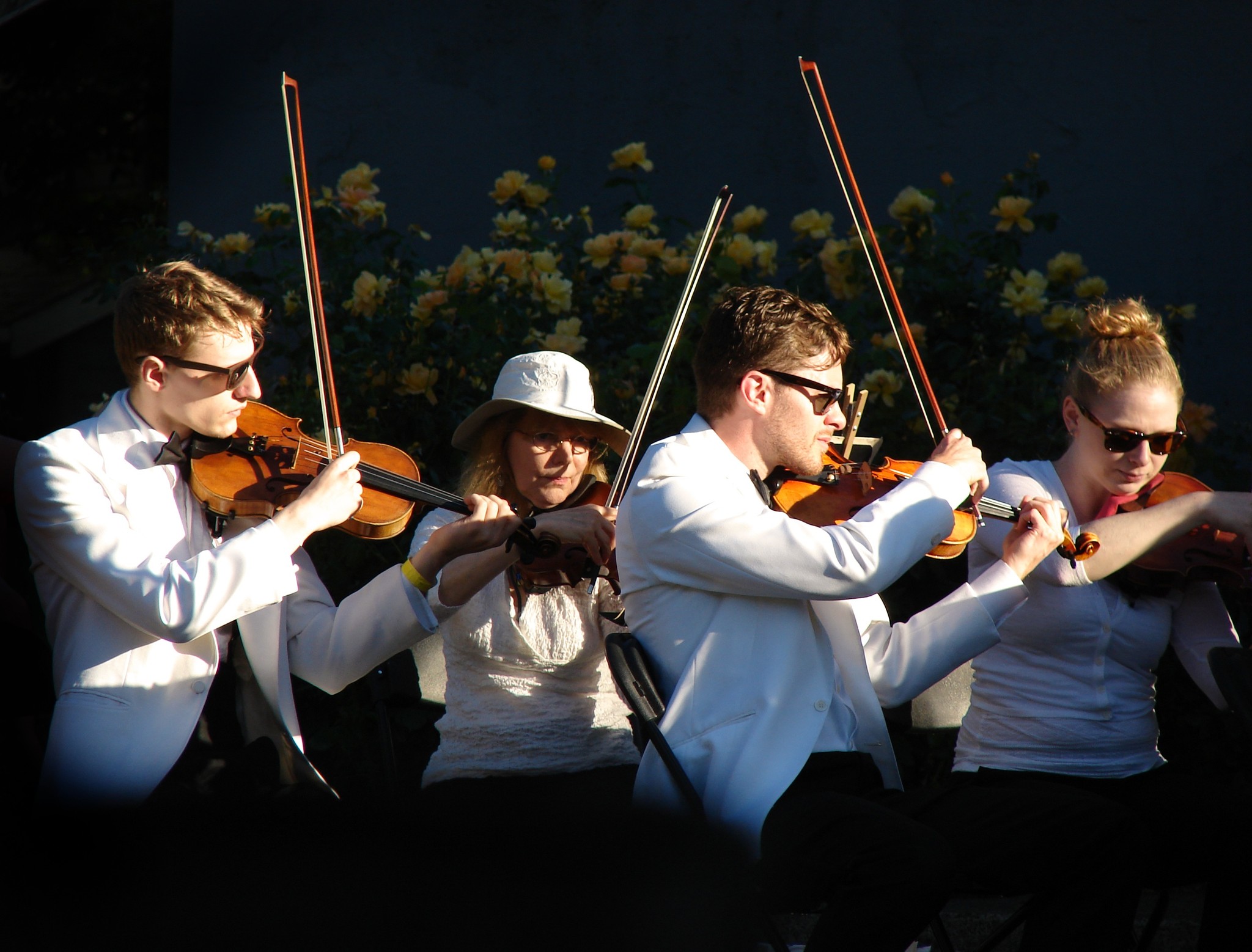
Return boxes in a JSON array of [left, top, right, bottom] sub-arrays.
[[451, 351, 633, 458]]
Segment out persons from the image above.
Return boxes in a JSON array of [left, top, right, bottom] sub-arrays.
[[618, 282, 1069, 952], [13, 256, 523, 952], [397, 347, 643, 952], [951, 298, 1252, 952]]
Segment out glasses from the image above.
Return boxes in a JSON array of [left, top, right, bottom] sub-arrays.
[[512, 425, 598, 453], [132, 327, 265, 390], [734, 367, 844, 415], [1074, 395, 1187, 456]]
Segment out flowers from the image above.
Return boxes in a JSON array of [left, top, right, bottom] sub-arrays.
[[167, 135, 1220, 519]]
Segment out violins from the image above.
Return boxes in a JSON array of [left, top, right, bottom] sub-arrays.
[[510, 475, 619, 584], [769, 443, 1100, 569], [188, 400, 563, 565], [1113, 471, 1252, 589]]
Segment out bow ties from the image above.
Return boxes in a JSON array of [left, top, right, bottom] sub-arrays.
[[750, 468, 774, 510], [152, 430, 193, 482]]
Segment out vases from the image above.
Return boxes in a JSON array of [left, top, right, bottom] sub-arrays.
[[394, 625, 471, 710], [902, 647, 986, 742]]
